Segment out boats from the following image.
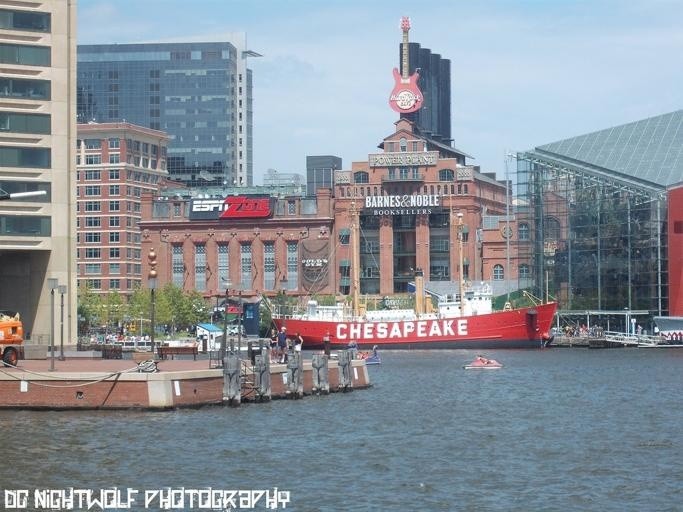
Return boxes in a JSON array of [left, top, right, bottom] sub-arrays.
[[547, 310, 682, 348], [463, 355, 503, 369], [272, 202, 559, 348]]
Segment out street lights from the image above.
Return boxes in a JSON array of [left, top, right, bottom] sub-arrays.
[[46, 277, 68, 371]]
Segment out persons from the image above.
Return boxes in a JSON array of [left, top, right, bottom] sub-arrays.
[[269, 326, 330, 364]]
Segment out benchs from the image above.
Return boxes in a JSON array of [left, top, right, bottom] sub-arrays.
[[132, 346, 199, 372]]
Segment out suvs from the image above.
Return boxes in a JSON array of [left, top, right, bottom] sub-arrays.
[[0, 311, 23, 366]]
